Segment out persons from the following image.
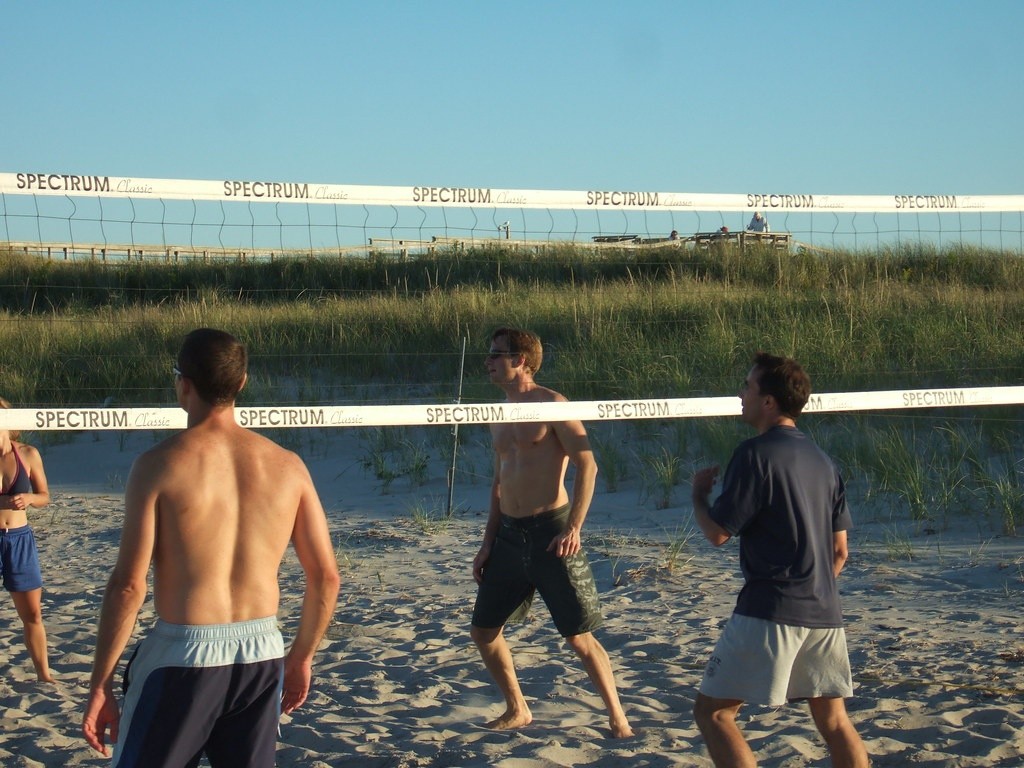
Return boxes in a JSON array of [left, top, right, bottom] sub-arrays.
[[668, 230, 681, 240], [82, 327, 340, 768], [690, 352, 870, 768], [0, 398, 59, 686], [747, 212, 770, 233], [715, 226, 728, 237], [470, 327, 635, 738]]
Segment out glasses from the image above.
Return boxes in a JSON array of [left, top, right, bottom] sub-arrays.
[[488, 350, 520, 360]]
